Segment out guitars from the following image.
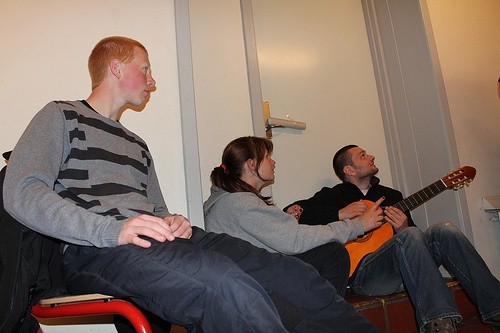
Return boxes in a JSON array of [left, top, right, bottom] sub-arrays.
[[344, 166, 476, 279]]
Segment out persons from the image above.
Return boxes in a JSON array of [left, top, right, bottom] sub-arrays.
[[282, 144, 500, 332], [202, 135, 385, 301], [2, 36, 387, 333]]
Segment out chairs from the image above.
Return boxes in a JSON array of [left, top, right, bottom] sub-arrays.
[[32, 293, 152, 333]]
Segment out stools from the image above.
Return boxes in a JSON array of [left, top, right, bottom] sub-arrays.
[[346, 279, 482, 333]]
